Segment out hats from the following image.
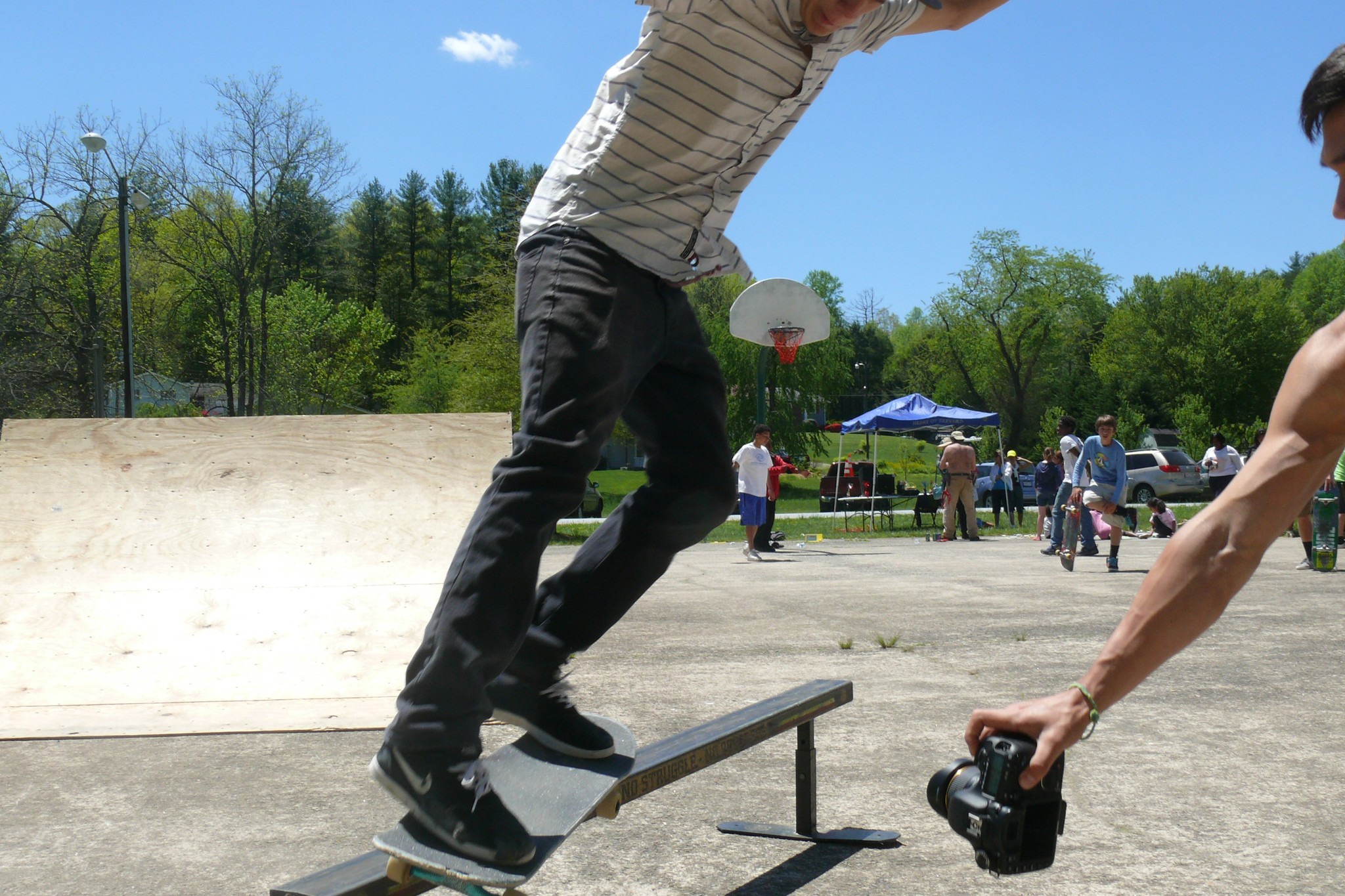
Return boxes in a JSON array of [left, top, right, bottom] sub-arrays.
[[1007, 450, 1017, 458], [937, 437, 953, 447], [951, 431, 965, 441]]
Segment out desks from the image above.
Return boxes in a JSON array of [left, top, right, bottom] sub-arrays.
[[838, 492, 933, 534]]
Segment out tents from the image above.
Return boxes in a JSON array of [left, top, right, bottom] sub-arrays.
[[833, 393, 1010, 534]]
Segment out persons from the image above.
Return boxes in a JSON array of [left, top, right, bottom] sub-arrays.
[[1295, 470, 1335, 570], [936, 437, 968, 539], [1319, 448, 1345, 548], [1007, 450, 1033, 527], [939, 431, 979, 541], [1071, 415, 1138, 572], [989, 450, 1015, 527], [368, 0, 1010, 867], [964, 41, 1345, 789], [1033, 447, 1065, 541], [1040, 416, 1099, 556], [753, 439, 813, 552], [732, 424, 776, 562], [1201, 433, 1243, 497], [1246, 428, 1267, 461], [1146, 497, 1178, 537], [1089, 508, 1149, 539]]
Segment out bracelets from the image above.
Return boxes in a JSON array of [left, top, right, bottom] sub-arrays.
[[1172, 532, 1174, 534], [1068, 684, 1099, 740]]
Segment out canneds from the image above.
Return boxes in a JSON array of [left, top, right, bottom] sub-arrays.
[[932, 533, 941, 541], [925, 533, 930, 542]]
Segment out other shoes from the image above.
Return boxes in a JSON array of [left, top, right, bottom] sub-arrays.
[[1153, 534, 1168, 538], [941, 536, 953, 541], [1033, 536, 1042, 541], [754, 544, 776, 552], [1138, 533, 1150, 540], [970, 536, 980, 541]]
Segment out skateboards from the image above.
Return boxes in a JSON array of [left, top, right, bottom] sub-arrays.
[[373, 712, 638, 896], [1055, 490, 1082, 572], [1311, 483, 1340, 572]]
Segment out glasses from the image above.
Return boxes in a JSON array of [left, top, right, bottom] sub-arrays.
[[760, 433, 771, 441]]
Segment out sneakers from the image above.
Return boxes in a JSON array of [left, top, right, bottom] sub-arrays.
[[1125, 507, 1139, 533], [1040, 545, 1061, 555], [742, 546, 750, 556], [1075, 546, 1099, 556], [1337, 539, 1345, 549], [369, 740, 537, 867], [747, 548, 762, 561], [491, 665, 616, 759], [1106, 555, 1119, 571], [1296, 558, 1313, 570]]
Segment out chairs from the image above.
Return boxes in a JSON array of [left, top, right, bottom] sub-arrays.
[[911, 493, 940, 529]]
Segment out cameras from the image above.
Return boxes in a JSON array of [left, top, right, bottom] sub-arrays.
[[927, 733, 1067, 875]]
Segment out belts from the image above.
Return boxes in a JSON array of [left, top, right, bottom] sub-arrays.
[[949, 473, 972, 476]]
